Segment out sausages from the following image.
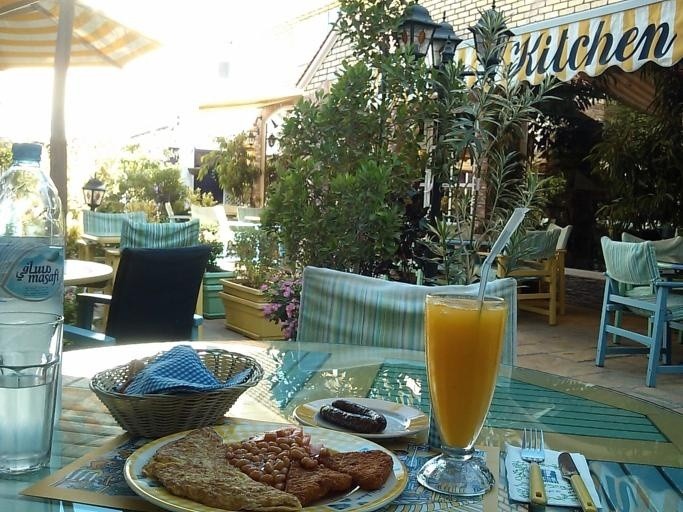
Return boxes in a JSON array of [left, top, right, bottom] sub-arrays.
[[320, 399, 387, 432]]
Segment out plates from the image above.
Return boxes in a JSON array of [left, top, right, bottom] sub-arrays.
[[122, 419, 410, 511], [291, 395, 431, 443]]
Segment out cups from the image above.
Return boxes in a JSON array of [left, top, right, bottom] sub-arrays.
[[0, 310, 66, 479]]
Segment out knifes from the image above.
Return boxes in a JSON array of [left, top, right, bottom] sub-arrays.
[[556, 450, 598, 512]]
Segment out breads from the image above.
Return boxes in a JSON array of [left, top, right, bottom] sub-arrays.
[[141, 425, 393, 508]]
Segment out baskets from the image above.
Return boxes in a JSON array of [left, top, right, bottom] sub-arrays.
[[87, 344, 266, 440]]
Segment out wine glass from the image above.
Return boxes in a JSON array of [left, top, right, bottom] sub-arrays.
[[414, 291, 510, 498]]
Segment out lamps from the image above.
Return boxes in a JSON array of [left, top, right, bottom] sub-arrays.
[[264, 134, 279, 148], [244, 127, 260, 145]]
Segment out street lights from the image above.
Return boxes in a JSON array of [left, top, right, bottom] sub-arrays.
[[390, 1, 515, 281]]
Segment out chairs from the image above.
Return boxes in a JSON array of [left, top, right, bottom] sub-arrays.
[[595, 232, 682, 387], [428, 219, 572, 326], [293, 265, 518, 364], [57, 202, 287, 346]]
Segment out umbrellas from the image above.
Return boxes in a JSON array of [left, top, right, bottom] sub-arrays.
[[0, 1, 162, 71]]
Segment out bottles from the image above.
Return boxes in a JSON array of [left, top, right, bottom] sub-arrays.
[[1, 140, 67, 433]]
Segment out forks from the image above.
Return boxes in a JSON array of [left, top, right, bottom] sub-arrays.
[[517, 426, 549, 509]]
